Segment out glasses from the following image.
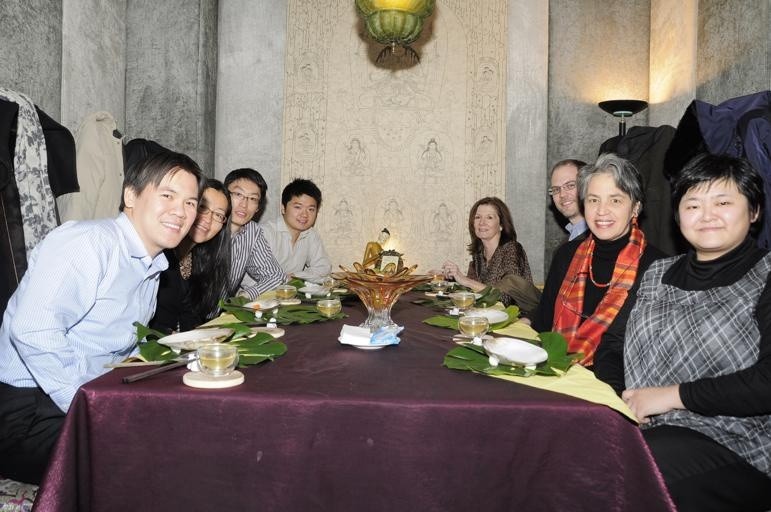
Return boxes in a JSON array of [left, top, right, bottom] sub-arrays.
[[198, 204, 227, 223], [229, 190, 262, 205], [548, 183, 576, 196], [560, 272, 611, 319]]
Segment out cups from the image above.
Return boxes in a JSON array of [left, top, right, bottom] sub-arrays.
[[428, 271, 444, 282]]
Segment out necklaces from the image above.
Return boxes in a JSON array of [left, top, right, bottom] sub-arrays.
[[590, 228, 648, 288]]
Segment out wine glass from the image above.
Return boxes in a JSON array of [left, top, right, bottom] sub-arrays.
[[329, 262, 433, 333]]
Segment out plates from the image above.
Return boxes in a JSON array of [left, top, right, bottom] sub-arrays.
[[158, 327, 235, 350], [182, 377, 252, 389], [450, 292, 483, 299], [243, 299, 280, 312], [462, 306, 510, 324], [279, 299, 303, 305], [297, 286, 326, 297], [484, 336, 548, 365]]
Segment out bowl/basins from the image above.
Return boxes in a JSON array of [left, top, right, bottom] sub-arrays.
[[458, 317, 490, 336], [454, 294, 475, 307], [275, 285, 298, 301], [431, 281, 449, 292], [196, 344, 241, 376], [321, 277, 342, 289], [317, 299, 343, 317]]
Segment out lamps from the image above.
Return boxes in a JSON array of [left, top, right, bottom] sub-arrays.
[[598, 100, 648, 136], [354, 0, 435, 70]]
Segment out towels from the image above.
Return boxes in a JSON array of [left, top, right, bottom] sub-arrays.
[[30, 276, 679, 512], [196, 312, 245, 329], [489, 364, 640, 427], [255, 291, 275, 306], [492, 322, 541, 341]]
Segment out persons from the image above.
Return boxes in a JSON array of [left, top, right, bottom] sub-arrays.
[[548, 159, 590, 243], [238, 177, 332, 298], [593, 149, 771, 511], [148, 178, 232, 341], [207, 167, 286, 318], [1, 152, 205, 490], [527, 154, 671, 374], [441, 196, 532, 309]]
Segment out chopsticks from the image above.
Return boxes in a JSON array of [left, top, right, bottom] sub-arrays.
[[123, 360, 196, 384], [485, 331, 541, 347], [302, 300, 354, 308], [198, 322, 266, 329]]
